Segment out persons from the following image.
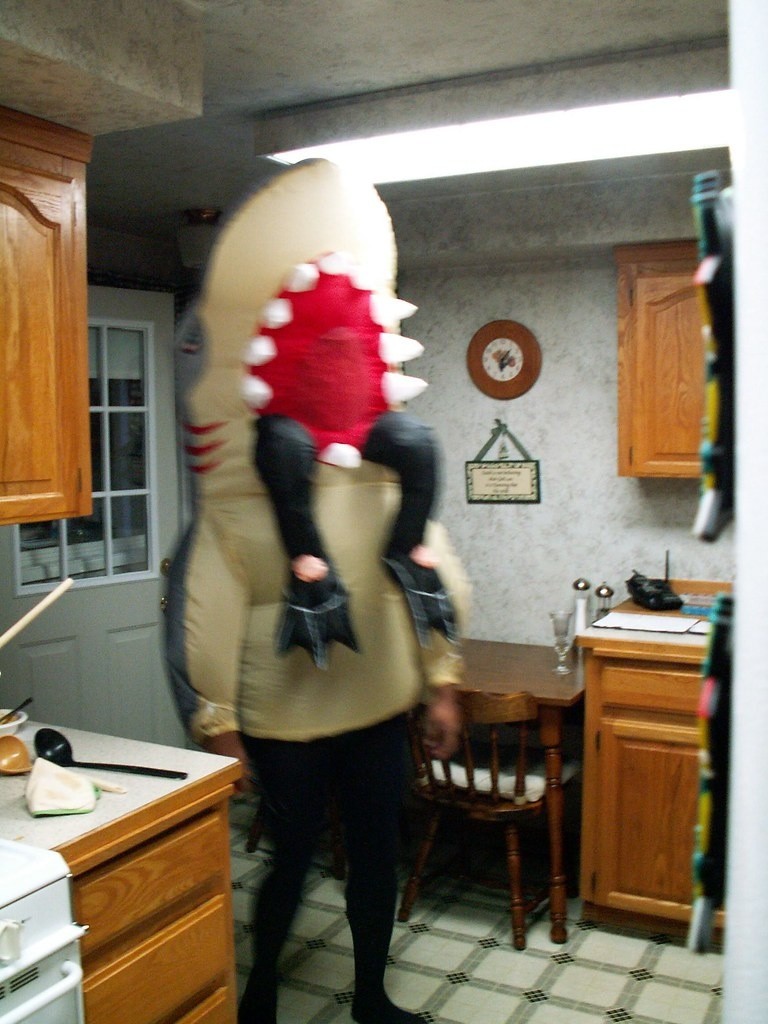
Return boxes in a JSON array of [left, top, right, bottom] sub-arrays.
[[162, 156, 469, 1024]]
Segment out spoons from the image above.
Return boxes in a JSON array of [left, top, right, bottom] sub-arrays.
[[0, 697, 34, 723]]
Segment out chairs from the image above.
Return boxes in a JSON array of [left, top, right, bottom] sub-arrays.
[[399, 689, 581, 949]]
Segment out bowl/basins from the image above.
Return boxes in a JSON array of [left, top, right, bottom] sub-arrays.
[[0, 709, 28, 737]]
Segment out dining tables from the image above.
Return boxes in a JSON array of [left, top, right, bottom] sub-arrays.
[[236, 636, 585, 946]]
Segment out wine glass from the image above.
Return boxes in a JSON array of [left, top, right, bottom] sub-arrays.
[[551, 614, 571, 677]]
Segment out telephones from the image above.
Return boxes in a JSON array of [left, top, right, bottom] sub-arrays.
[[625, 569, 683, 611]]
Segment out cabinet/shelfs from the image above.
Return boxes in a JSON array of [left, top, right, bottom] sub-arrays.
[[615, 240, 712, 478], [571, 578, 734, 945], [2, 720, 245, 1023], [1, 109, 93, 528]]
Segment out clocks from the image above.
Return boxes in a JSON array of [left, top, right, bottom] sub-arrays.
[[465, 319, 543, 401]]
